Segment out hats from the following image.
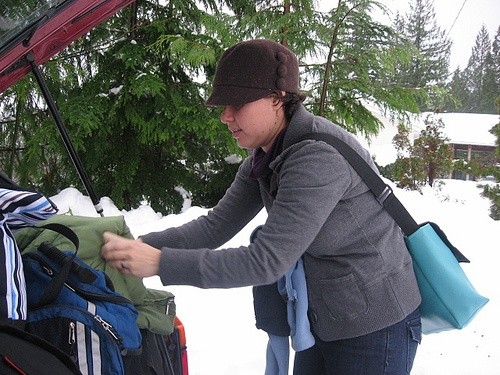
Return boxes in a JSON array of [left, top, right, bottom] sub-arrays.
[[204, 39, 300, 106]]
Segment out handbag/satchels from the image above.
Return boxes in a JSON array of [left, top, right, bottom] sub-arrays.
[[0, 188, 190, 374], [404, 222, 490, 335]]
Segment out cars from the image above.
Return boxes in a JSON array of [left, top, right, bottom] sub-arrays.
[[0, 0, 191, 375]]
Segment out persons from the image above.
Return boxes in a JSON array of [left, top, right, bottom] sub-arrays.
[[101, 40, 423, 375]]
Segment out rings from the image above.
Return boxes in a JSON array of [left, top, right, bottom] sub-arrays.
[[120, 260, 127, 269]]
